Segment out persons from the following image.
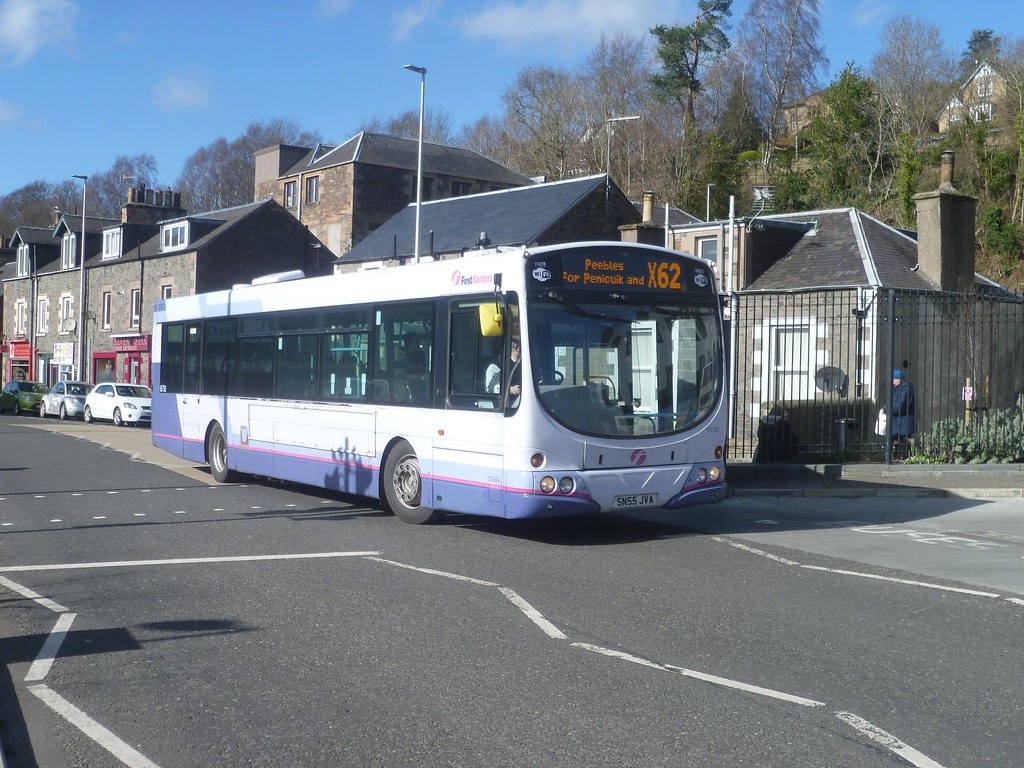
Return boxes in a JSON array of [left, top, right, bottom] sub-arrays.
[[880, 369, 918, 461], [393, 331, 431, 406], [485, 350, 502, 394], [499, 335, 523, 410]]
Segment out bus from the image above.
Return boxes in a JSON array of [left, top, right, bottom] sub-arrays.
[[149, 239, 728, 528]]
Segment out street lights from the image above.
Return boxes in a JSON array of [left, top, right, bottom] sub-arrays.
[[71, 174, 89, 379], [403, 63, 428, 263]]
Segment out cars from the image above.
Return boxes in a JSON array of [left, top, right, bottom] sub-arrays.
[[1, 380, 49, 415], [38, 380, 95, 420], [83, 382, 154, 426]]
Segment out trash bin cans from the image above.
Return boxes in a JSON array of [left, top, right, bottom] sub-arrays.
[[835, 417, 860, 464]]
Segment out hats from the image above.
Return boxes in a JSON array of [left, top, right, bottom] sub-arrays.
[[892, 369, 904, 378]]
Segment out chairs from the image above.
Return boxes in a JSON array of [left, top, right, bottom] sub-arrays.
[[161, 344, 624, 436]]
